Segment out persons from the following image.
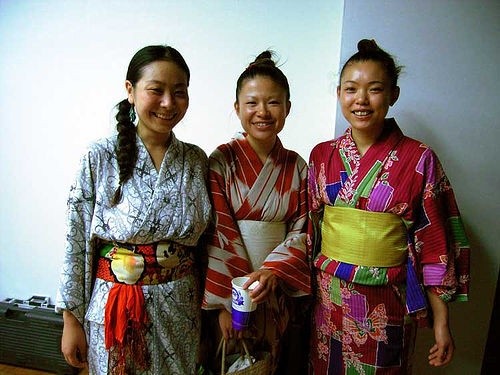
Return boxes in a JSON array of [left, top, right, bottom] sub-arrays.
[[54, 44, 213, 375], [200, 44, 312, 375], [305, 37, 472, 375]]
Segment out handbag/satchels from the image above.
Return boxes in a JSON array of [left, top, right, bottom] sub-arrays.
[[205, 350, 272, 375]]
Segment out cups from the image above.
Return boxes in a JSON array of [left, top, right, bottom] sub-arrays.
[[231, 276, 260, 330]]
